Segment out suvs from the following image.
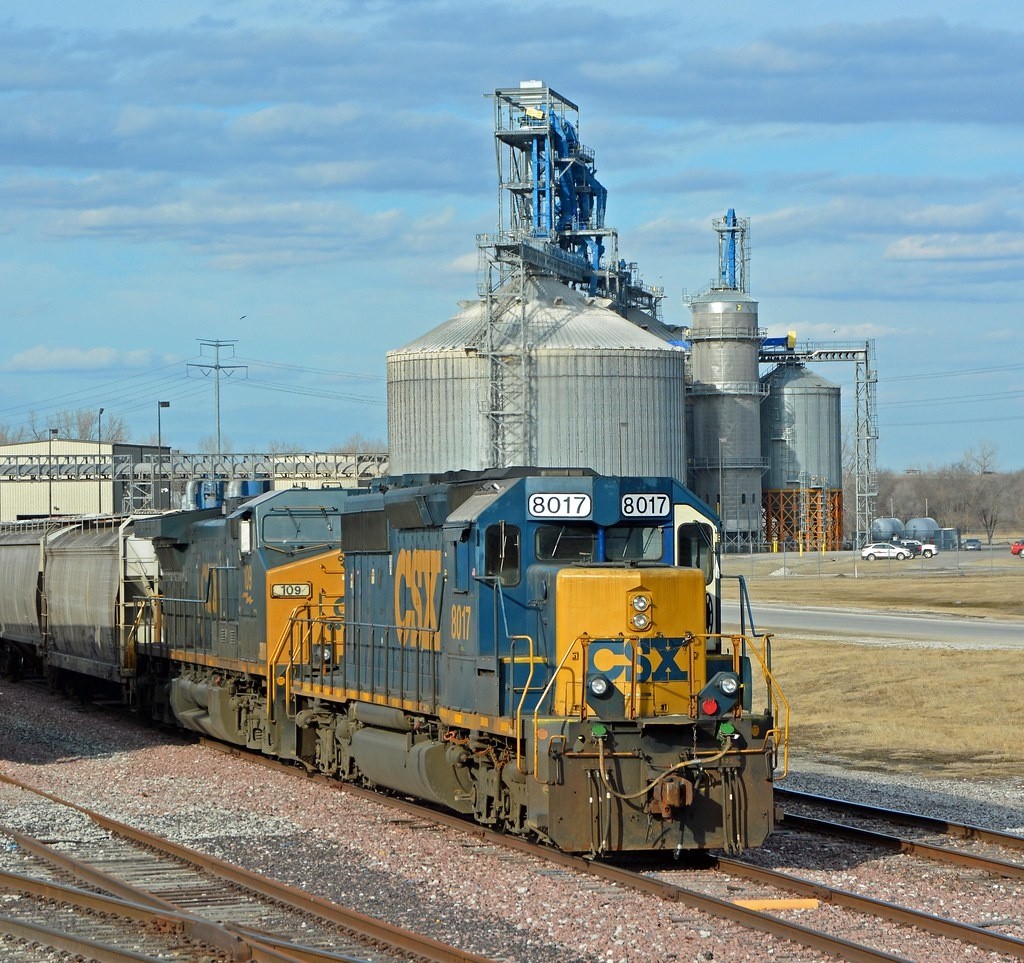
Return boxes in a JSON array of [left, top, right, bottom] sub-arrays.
[[902, 540, 939, 558], [862, 543, 911, 561]]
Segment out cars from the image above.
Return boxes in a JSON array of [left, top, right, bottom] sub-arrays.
[[965, 539, 981, 551], [1011, 540, 1024, 558], [892, 542, 922, 559]]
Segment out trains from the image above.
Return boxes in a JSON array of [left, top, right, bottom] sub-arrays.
[[0, 467, 789, 860]]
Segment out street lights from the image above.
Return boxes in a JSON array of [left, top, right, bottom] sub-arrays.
[[157, 401, 170, 510], [49, 428, 58, 518], [99, 408, 104, 513]]
